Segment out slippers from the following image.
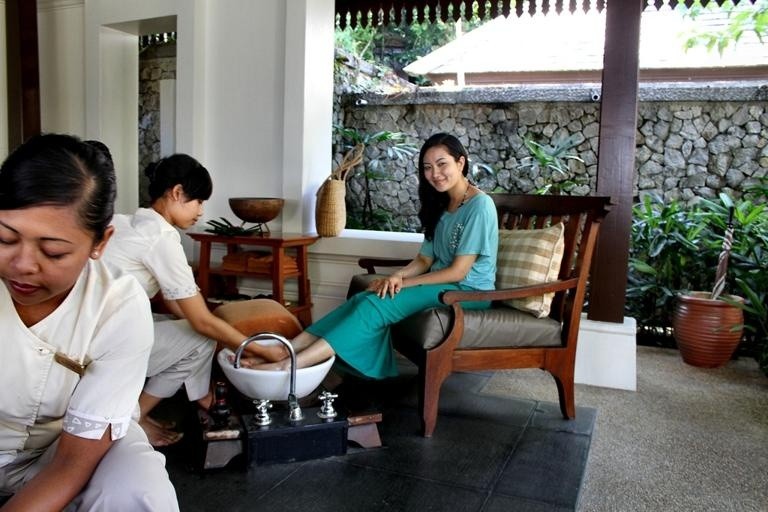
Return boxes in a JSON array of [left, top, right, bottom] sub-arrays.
[[224, 295, 253, 304], [207, 295, 223, 303], [253, 294, 291, 306]]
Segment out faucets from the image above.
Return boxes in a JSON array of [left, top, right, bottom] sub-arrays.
[[233, 331, 306, 422]]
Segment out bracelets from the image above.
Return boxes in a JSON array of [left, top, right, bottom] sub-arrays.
[[392, 270, 407, 275]]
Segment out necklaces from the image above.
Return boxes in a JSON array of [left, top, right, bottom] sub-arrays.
[[455, 183, 470, 208]]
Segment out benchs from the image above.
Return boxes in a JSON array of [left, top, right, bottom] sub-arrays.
[[346, 192, 619, 439]]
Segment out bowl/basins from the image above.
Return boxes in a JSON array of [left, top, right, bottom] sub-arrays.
[[229, 197, 285, 223]]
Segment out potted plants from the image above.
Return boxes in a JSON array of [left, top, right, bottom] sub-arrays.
[[672, 196, 764, 369]]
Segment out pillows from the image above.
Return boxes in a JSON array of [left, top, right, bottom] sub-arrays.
[[497, 216, 567, 318]]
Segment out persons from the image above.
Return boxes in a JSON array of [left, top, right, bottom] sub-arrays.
[[0, 131, 184, 511], [228, 132, 499, 385], [99, 152, 287, 448]]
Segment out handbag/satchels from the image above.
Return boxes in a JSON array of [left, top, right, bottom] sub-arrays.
[[316, 143, 365, 237]]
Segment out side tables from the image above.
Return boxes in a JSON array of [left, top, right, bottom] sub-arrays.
[[187, 226, 321, 328]]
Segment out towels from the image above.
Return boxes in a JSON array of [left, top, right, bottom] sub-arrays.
[[221, 252, 257, 272], [247, 255, 299, 274]]
